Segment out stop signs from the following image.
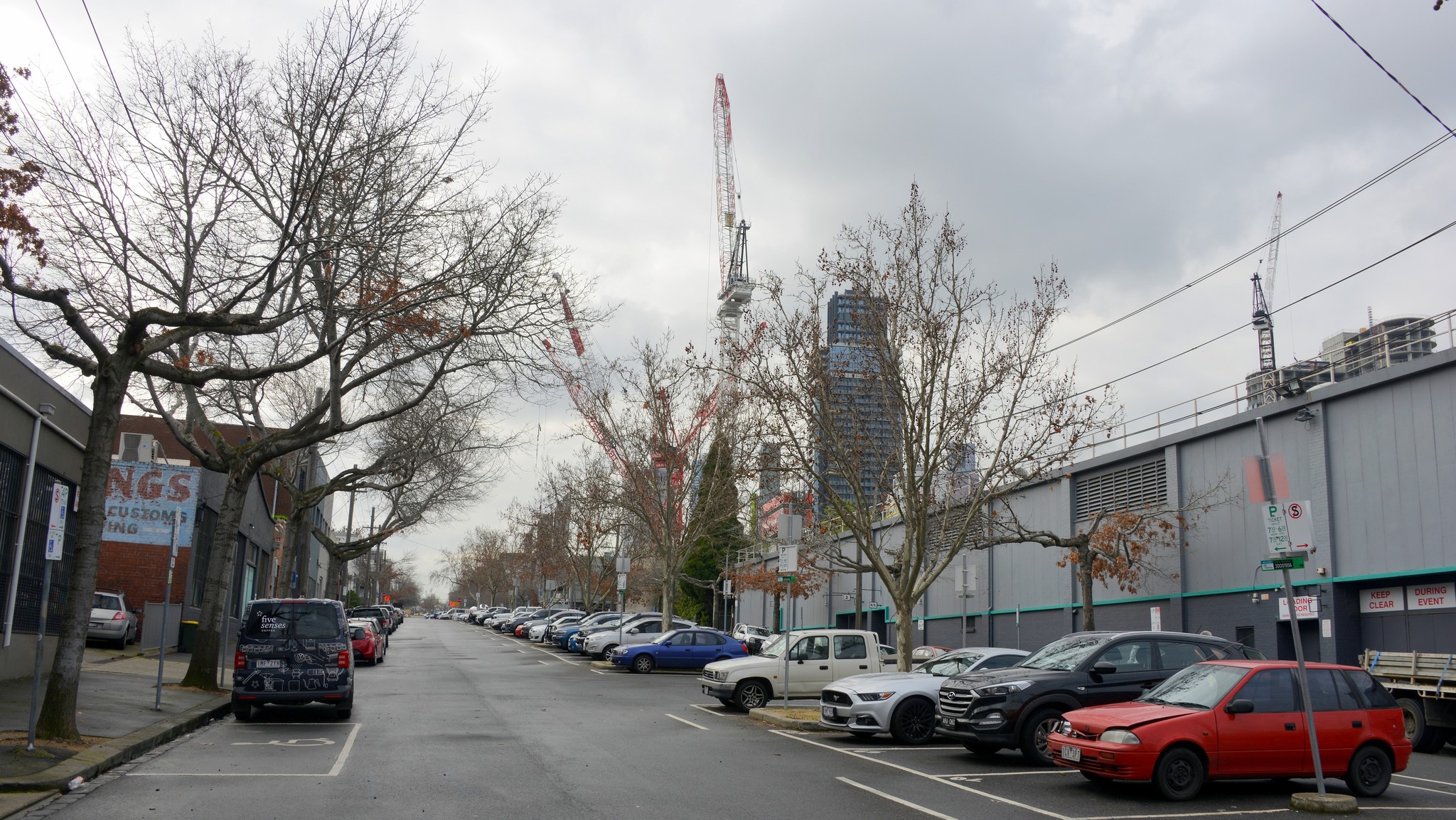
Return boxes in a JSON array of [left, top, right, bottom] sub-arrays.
[[381, 592, 386, 597]]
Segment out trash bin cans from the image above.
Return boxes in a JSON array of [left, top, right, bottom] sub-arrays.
[[176, 620, 199, 654]]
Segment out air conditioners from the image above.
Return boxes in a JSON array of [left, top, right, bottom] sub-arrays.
[[118, 432, 169, 464]]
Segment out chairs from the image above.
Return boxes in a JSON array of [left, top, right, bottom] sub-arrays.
[[684, 635, 691, 644], [809, 646, 823, 658], [688, 636, 699, 645], [1104, 647, 1124, 672], [1135, 645, 1148, 670], [819, 646, 828, 658]]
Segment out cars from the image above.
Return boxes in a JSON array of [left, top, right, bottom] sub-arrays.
[[1045, 658, 1414, 798], [912, 645, 956, 659], [370, 603, 406, 635], [85, 587, 142, 650], [875, 643, 898, 655], [814, 646, 1033, 746], [412, 606, 682, 657], [348, 607, 389, 647], [582, 616, 702, 662], [347, 617, 387, 655], [610, 630, 750, 675], [232, 597, 354, 718], [343, 621, 386, 664]]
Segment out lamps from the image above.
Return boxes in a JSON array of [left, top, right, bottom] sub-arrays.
[[1293, 407, 1318, 422], [1273, 377, 1306, 399]]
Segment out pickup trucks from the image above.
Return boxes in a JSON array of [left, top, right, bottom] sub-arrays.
[[702, 628, 934, 712]]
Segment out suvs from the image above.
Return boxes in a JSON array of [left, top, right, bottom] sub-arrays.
[[931, 628, 1271, 766]]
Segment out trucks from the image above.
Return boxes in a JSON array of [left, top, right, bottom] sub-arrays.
[[1357, 648, 1456, 755], [718, 623, 773, 654]]
